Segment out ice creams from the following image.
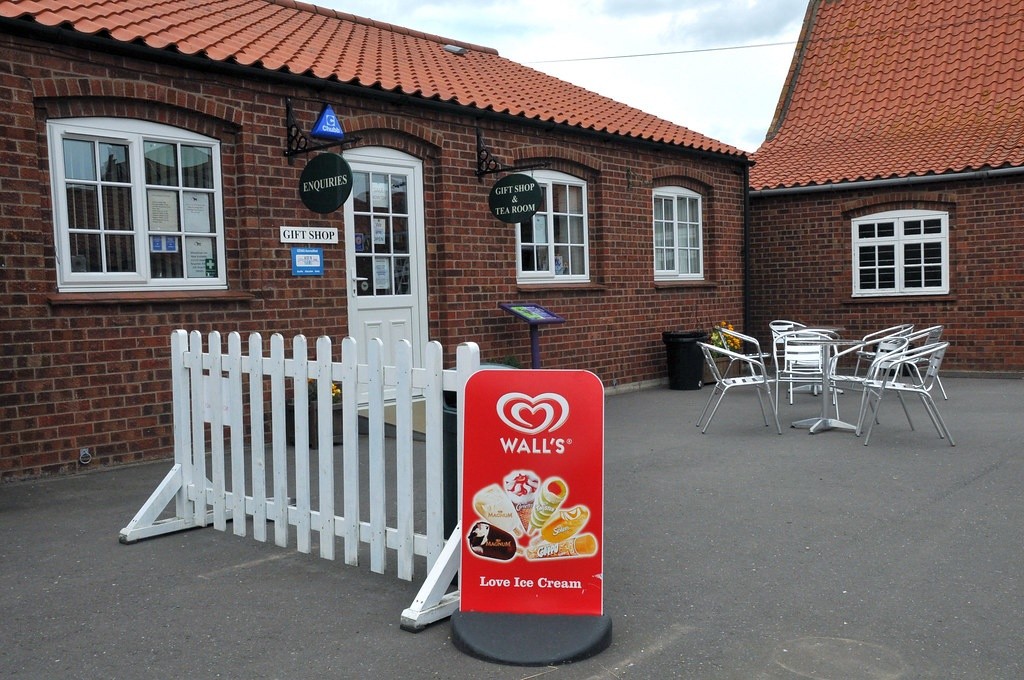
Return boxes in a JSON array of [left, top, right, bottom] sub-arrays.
[[503, 469, 540, 531]]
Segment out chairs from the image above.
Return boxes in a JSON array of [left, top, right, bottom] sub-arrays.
[[789, 329, 840, 405], [770, 320, 827, 387], [696, 341, 782, 435], [773, 332, 842, 421], [851, 324, 915, 390], [714, 325, 772, 394], [885, 325, 948, 401], [828, 337, 915, 434], [857, 341, 956, 447]]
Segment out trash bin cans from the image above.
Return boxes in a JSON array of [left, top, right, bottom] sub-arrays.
[[874, 342, 915, 377], [662, 330, 710, 392], [442, 362, 521, 587]]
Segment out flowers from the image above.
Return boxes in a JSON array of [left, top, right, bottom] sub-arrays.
[[706, 321, 743, 358], [308, 378, 342, 404]]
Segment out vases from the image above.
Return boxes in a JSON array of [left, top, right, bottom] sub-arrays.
[[703, 356, 742, 383], [287, 398, 343, 450]]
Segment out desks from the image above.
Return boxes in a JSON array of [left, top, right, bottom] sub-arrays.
[[787, 326, 846, 395], [787, 339, 866, 434]]
